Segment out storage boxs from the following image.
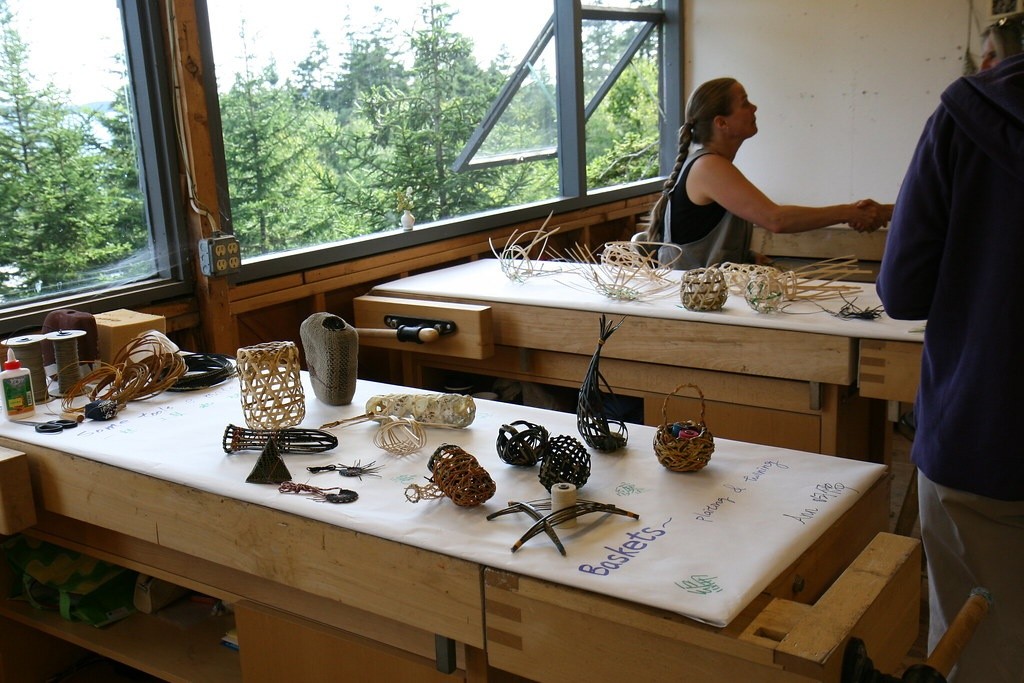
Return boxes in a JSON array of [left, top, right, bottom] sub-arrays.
[[92, 308, 166, 366]]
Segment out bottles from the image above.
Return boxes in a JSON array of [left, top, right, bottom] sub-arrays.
[[0, 347, 36, 420]]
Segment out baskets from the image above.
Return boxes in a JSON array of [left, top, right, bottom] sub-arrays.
[[238, 339, 305, 432], [681, 268, 728, 313], [427, 443, 496, 508], [537, 435, 591, 495], [654, 383, 714, 472]]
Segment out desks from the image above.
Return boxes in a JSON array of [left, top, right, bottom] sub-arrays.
[[0, 351, 920, 683], [352, 258, 928, 457], [635, 216, 892, 284]]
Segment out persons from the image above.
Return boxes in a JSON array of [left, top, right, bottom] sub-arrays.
[[647, 78, 888, 271], [852, 0, 1024, 682]]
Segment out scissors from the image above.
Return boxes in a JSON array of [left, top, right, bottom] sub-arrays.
[[12, 418, 80, 435]]
